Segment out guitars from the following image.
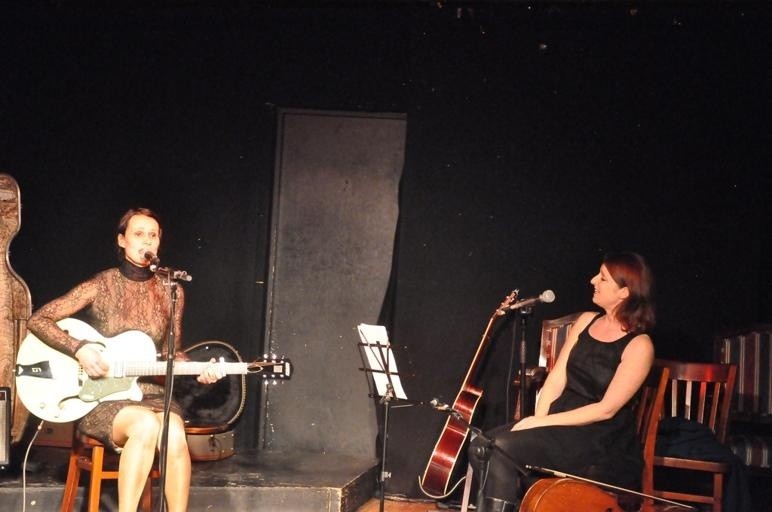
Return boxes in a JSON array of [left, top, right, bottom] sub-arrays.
[[13, 317, 294, 424], [420, 287, 520, 498]]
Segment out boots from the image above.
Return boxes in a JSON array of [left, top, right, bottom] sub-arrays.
[[484, 495, 517, 512]]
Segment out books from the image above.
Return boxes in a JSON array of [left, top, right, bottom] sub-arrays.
[[357, 322, 407, 399]]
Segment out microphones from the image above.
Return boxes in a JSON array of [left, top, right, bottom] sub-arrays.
[[144, 251, 193, 283], [497, 288, 556, 316]]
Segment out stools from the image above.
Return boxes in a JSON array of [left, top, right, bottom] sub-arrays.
[[60, 427, 164, 511]]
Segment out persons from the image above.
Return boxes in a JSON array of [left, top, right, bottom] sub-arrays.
[[467, 251, 656, 512], [25, 207, 228, 512]]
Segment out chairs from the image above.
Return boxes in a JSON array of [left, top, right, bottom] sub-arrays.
[[629, 357, 671, 511], [720, 330, 772, 470], [513, 311, 586, 426], [652, 362, 739, 512]]
